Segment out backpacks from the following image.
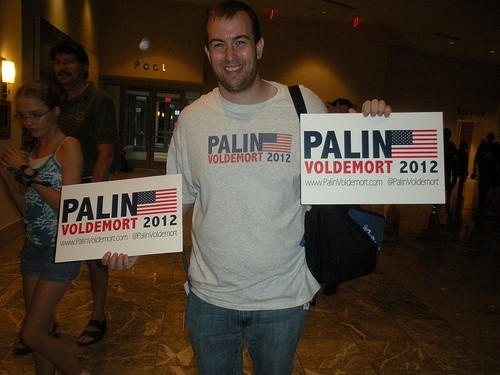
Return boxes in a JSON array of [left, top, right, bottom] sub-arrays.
[[300, 204, 385, 284]]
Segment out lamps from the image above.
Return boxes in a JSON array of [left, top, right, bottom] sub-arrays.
[[0, 57, 15, 97]]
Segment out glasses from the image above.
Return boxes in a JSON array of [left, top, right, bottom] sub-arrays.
[[14, 109, 50, 121]]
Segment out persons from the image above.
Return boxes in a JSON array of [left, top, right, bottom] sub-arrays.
[[0, 80, 90, 375], [429, 128, 500, 220], [14, 40, 115, 355], [102, 0, 391, 375]]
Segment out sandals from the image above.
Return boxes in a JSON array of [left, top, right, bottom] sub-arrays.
[[14, 321, 60, 355], [77, 317, 107, 346]]
